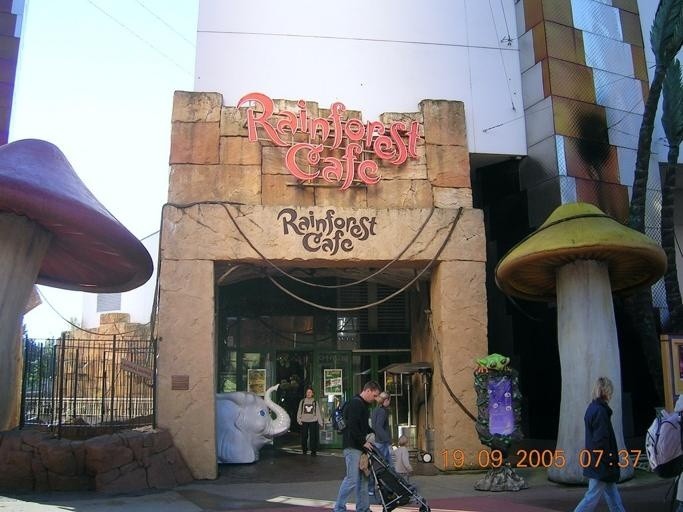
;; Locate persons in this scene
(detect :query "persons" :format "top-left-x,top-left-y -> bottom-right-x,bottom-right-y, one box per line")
572,375 -> 626,512
327,393 -> 339,421
392,434 -> 413,484
671,391 -> 683,511
296,387 -> 324,457
332,381 -> 382,512
368,392 -> 394,496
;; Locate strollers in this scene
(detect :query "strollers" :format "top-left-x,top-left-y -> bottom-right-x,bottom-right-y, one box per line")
363,444 -> 433,512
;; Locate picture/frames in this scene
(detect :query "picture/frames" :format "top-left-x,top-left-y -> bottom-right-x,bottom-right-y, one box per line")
323,368 -> 343,396
246,368 -> 267,397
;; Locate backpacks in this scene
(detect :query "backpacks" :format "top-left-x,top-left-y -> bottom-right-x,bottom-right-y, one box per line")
643,406 -> 682,504
328,396 -> 363,434
